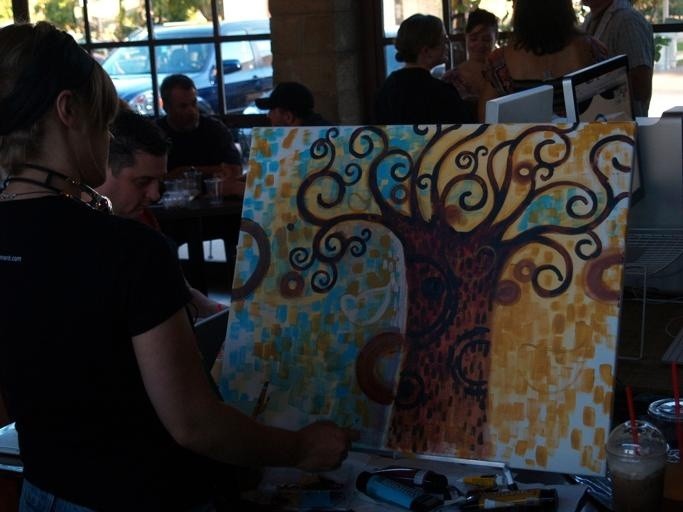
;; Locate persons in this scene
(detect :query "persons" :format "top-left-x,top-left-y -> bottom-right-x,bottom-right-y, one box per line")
442,9 -> 498,99
374,13 -> 478,124
255,81 -> 337,125
154,75 -> 241,165
482,0 -> 608,94
80,113 -> 230,319
578,0 -> 655,104
0,22 -> 362,512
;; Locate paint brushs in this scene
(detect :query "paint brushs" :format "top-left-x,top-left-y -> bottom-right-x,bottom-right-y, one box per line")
251,380 -> 270,420
342,419 -> 359,430
349,448 -> 401,459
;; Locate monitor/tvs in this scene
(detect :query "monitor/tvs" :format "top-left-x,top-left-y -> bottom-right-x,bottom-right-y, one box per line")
562,52 -> 646,211
483,83 -> 554,124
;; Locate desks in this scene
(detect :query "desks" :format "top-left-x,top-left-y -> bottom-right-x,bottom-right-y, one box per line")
149,165 -> 248,261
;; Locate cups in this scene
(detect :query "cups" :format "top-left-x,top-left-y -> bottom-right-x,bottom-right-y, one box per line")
662,450 -> 683,511
648,397 -> 683,449
604,419 -> 669,512
164,170 -> 225,207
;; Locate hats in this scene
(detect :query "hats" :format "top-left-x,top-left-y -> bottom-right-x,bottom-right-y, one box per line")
254,81 -> 314,110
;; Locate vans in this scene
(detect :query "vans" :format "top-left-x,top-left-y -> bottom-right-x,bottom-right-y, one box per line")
99,18 -> 275,117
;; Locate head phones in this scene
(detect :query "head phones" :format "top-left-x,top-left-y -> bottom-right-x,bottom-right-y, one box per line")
3,162 -> 114,215
